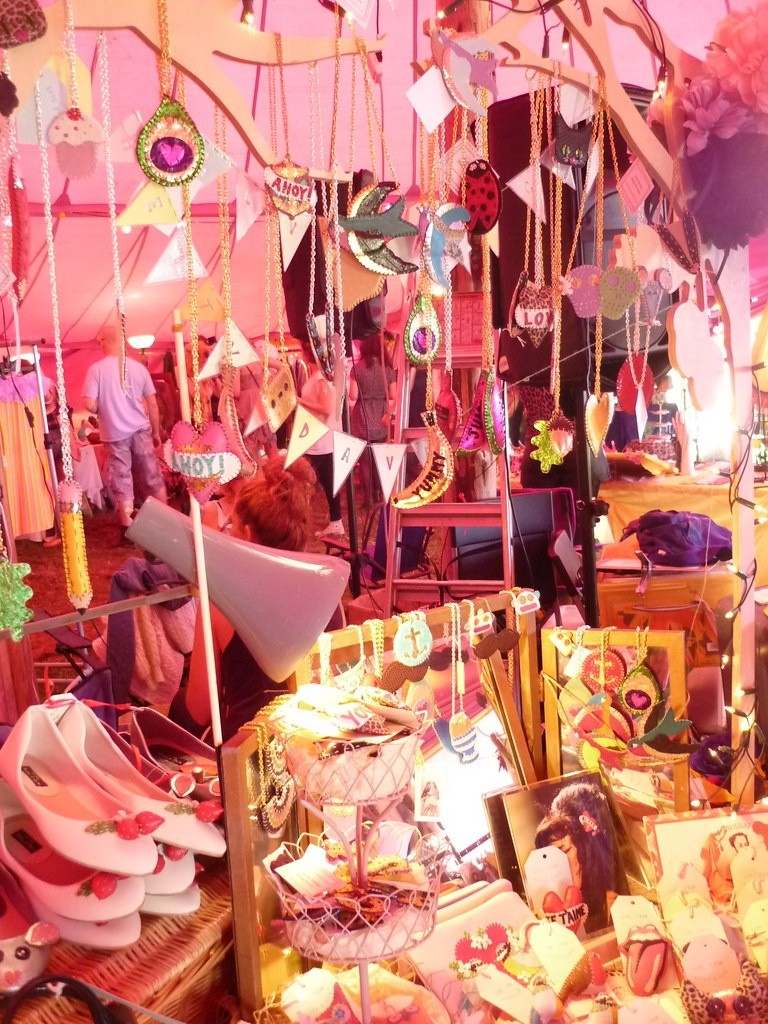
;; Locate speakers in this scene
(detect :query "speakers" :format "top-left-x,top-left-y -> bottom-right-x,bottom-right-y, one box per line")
487,76 -> 682,390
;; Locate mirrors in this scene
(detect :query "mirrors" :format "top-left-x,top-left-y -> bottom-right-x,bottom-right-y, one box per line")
292,608 -> 535,894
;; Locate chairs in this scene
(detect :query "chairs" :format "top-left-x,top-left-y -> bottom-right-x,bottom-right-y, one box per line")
320,487 -> 585,627
0,611 -> 116,751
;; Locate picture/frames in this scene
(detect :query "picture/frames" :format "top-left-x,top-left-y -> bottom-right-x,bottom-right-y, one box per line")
641,805 -> 768,999
223,694 -> 322,1024
484,770 -> 630,941
544,630 -> 690,821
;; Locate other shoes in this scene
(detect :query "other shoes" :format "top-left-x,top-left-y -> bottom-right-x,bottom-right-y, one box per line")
0,692 -> 225,999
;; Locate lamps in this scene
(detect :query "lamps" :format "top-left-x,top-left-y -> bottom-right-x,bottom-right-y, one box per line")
128,335 -> 155,352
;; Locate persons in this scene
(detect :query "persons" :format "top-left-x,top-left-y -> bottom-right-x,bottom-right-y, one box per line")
538,785 -> 621,934
0,334 -> 394,547
105,454 -> 330,748
604,375 -> 671,451
